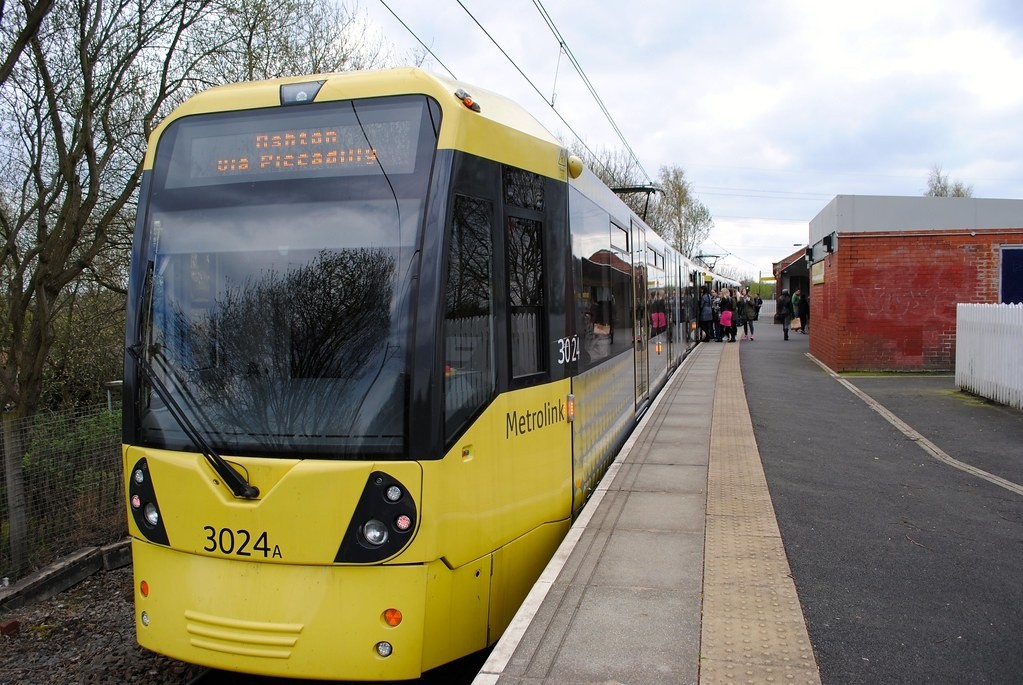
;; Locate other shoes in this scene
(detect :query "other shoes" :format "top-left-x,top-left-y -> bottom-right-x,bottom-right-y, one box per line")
702,340 -> 709,342
715,340 -> 722,342
729,340 -> 735,342
784,335 -> 788,340
750,335 -> 754,340
744,335 -> 747,340
800,330 -> 806,334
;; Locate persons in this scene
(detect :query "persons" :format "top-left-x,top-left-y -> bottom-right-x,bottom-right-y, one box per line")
700,286 -> 762,343
777,289 -> 795,341
607,294 -> 615,344
632,286 -> 695,351
583,312 -> 609,365
791,288 -> 810,335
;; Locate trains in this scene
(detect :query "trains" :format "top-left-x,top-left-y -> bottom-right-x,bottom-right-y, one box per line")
117,68 -> 745,685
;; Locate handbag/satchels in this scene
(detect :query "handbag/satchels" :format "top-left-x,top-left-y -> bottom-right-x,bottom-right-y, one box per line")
774,312 -> 782,320
791,318 -> 801,330
